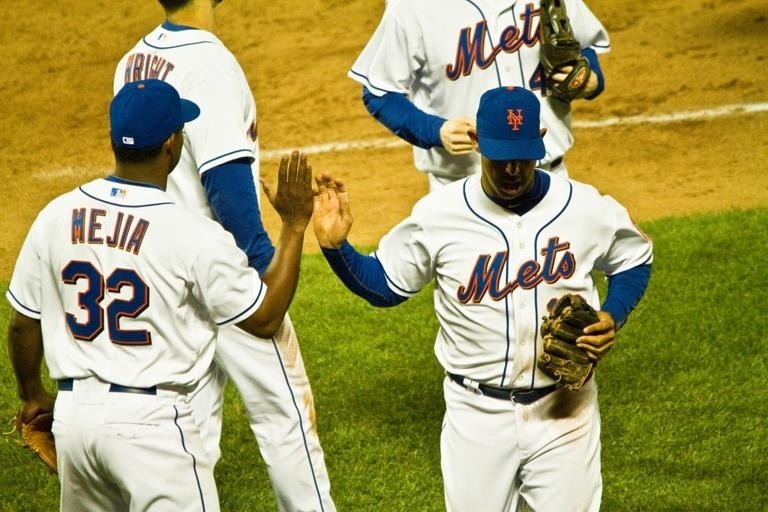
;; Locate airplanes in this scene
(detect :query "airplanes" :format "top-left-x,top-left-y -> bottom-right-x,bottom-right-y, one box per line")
2,409 -> 57,473
536,293 -> 600,391
539,0 -> 590,104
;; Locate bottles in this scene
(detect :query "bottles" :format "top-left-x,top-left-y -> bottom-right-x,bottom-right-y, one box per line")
109,79 -> 201,148
477,86 -> 546,162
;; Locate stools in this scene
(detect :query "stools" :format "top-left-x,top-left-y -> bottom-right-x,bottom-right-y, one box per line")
57,377 -> 158,395
447,371 -> 558,403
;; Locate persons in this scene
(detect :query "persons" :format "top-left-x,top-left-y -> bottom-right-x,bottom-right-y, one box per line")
110,0 -> 338,512
3,76 -> 314,512
343,0 -> 611,193
310,85 -> 654,512
